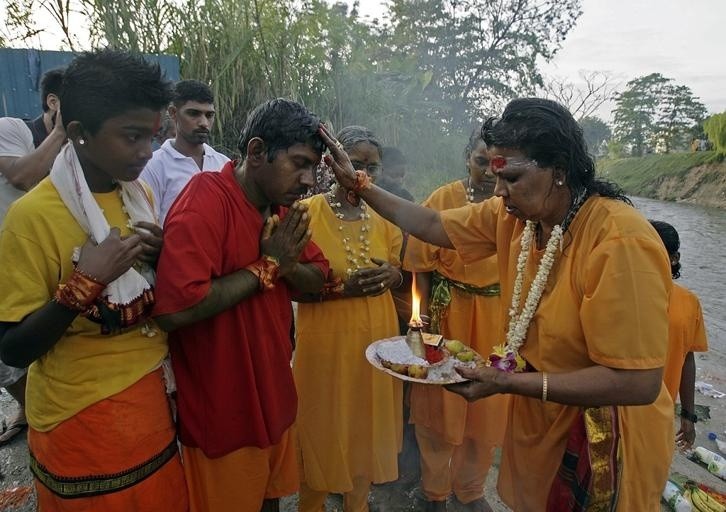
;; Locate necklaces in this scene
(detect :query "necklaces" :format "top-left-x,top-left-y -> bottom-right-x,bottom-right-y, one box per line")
465,176 -> 474,205
329,182 -> 370,281
485,187 -> 588,373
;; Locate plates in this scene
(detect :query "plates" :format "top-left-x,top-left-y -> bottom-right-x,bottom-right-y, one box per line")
362,332 -> 490,384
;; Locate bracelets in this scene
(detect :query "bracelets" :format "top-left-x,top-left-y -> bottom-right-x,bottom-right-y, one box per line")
345,170 -> 370,208
244,253 -> 280,292
320,267 -> 345,300
542,370 -> 549,404
391,271 -> 404,289
420,315 -> 431,325
680,408 -> 697,423
54,268 -> 106,312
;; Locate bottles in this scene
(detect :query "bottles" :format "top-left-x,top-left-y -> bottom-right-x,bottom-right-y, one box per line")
695,433 -> 726,479
404,321 -> 428,361
661,479 -> 690,508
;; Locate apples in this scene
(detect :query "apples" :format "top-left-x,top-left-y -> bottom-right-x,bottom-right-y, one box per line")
392,340 -> 475,379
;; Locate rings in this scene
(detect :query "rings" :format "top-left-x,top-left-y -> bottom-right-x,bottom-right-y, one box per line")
335,141 -> 344,152
380,283 -> 385,289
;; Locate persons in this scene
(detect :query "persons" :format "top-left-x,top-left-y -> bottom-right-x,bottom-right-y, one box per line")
150,98 -> 330,511
291,125 -> 404,512
373,146 -> 415,204
691,134 -> 707,152
0,47 -> 191,512
137,79 -> 232,231
402,129 -> 513,511
319,96 -> 676,511
647,218 -> 708,453
0,66 -> 70,444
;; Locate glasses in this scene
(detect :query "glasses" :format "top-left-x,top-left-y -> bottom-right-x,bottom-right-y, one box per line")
347,163 -> 383,178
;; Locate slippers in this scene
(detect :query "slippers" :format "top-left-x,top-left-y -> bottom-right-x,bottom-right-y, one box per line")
0,416 -> 30,446
